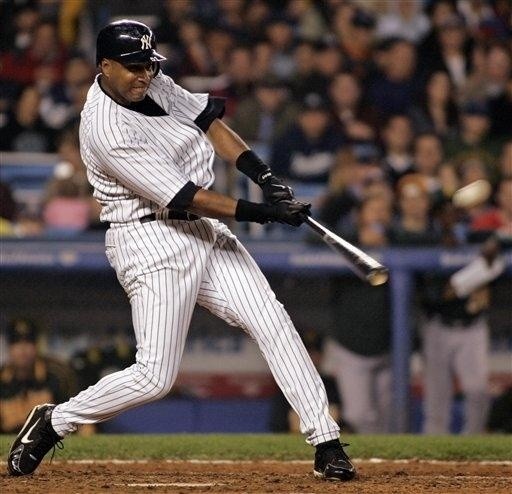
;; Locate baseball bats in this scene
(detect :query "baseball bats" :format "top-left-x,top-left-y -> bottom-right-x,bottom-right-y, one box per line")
303,213 -> 389,289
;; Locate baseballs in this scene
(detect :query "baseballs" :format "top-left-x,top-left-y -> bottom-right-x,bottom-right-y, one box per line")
453,179 -> 493,210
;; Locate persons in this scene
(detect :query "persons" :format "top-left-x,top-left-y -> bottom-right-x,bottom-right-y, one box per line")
7,20 -> 354,481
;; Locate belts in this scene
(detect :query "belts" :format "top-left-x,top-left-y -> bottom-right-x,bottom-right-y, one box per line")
139,211 -> 199,224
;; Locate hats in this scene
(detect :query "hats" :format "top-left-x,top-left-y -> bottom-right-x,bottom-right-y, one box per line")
96,20 -> 167,68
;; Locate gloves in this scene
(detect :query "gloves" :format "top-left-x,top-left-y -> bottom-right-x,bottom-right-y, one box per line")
236,150 -> 312,227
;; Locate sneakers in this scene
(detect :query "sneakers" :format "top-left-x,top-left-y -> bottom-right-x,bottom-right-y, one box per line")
313,438 -> 356,481
8,404 -> 63,476
